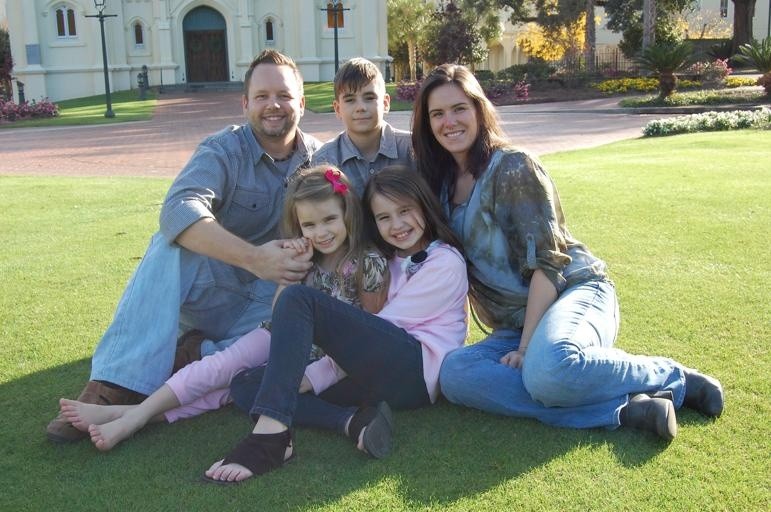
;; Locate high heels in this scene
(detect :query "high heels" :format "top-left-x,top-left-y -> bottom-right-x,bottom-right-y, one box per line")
627,391 -> 678,439
683,368 -> 723,417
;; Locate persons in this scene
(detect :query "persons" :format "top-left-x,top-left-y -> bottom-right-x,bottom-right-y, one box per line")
202,157 -> 472,486
308,55 -> 420,201
43,46 -> 326,446
409,58 -> 725,442
58,159 -> 393,456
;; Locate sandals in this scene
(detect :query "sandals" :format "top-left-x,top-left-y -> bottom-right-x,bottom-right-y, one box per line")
348,401 -> 393,459
203,430 -> 295,483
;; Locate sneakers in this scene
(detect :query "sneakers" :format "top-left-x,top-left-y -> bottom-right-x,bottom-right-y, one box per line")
46,381 -> 137,443
172,329 -> 208,375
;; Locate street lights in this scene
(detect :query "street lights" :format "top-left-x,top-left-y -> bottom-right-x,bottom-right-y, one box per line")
330,0 -> 341,76
92,0 -> 116,118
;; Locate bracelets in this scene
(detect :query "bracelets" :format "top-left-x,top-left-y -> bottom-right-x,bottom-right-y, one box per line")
517,346 -> 527,352
